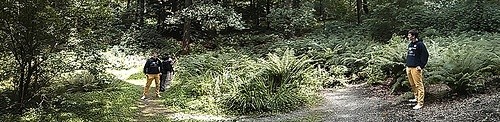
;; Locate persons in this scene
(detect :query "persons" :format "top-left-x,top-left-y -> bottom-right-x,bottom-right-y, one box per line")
143,51 -> 163,100
405,29 -> 428,110
157,53 -> 176,92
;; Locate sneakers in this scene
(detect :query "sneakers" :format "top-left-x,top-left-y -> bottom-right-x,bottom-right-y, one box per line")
413,103 -> 424,110
409,98 -> 418,102
156,94 -> 162,98
141,95 -> 146,100
